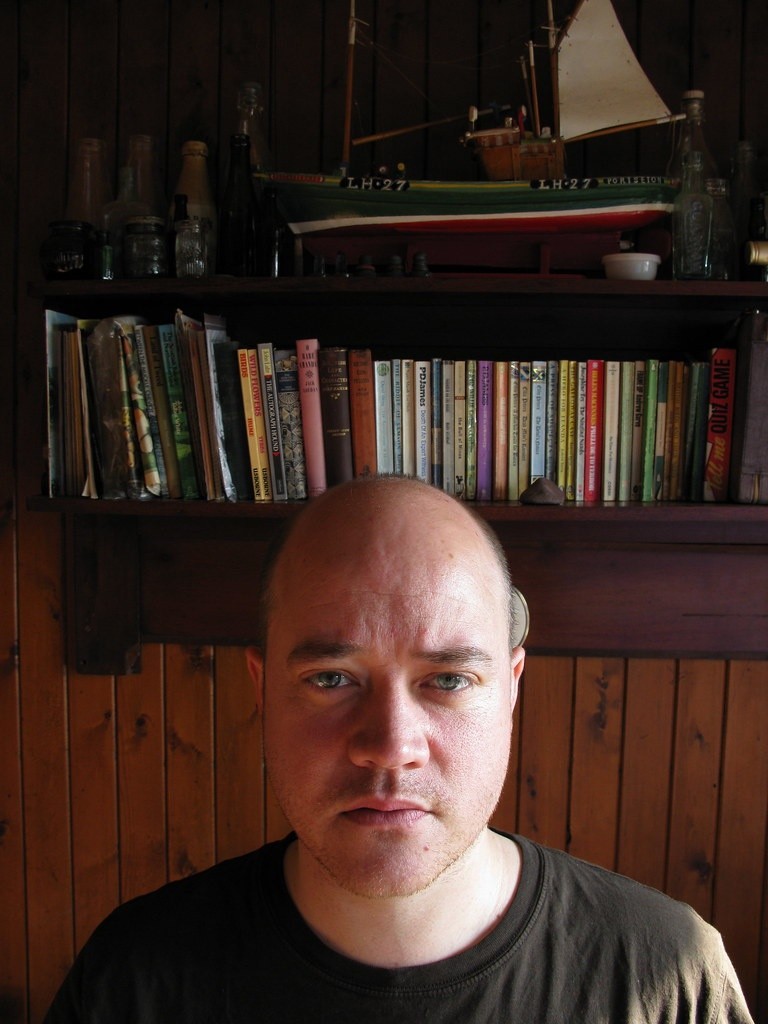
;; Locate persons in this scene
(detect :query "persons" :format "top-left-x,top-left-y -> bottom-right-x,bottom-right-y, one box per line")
40,473 -> 754,1024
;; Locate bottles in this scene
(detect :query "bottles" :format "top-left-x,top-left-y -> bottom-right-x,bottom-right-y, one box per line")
111,133 -> 167,276
55,136 -> 120,282
672,88 -> 721,279
169,141 -> 221,278
215,83 -> 275,276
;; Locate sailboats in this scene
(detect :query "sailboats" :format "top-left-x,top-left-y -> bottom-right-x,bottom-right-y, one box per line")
259,1 -> 688,273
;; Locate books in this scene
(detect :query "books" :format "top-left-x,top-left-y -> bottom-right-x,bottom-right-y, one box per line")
46,309 -> 735,502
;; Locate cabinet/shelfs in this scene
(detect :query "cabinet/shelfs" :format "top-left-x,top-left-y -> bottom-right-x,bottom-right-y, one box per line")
22,278 -> 768,675
